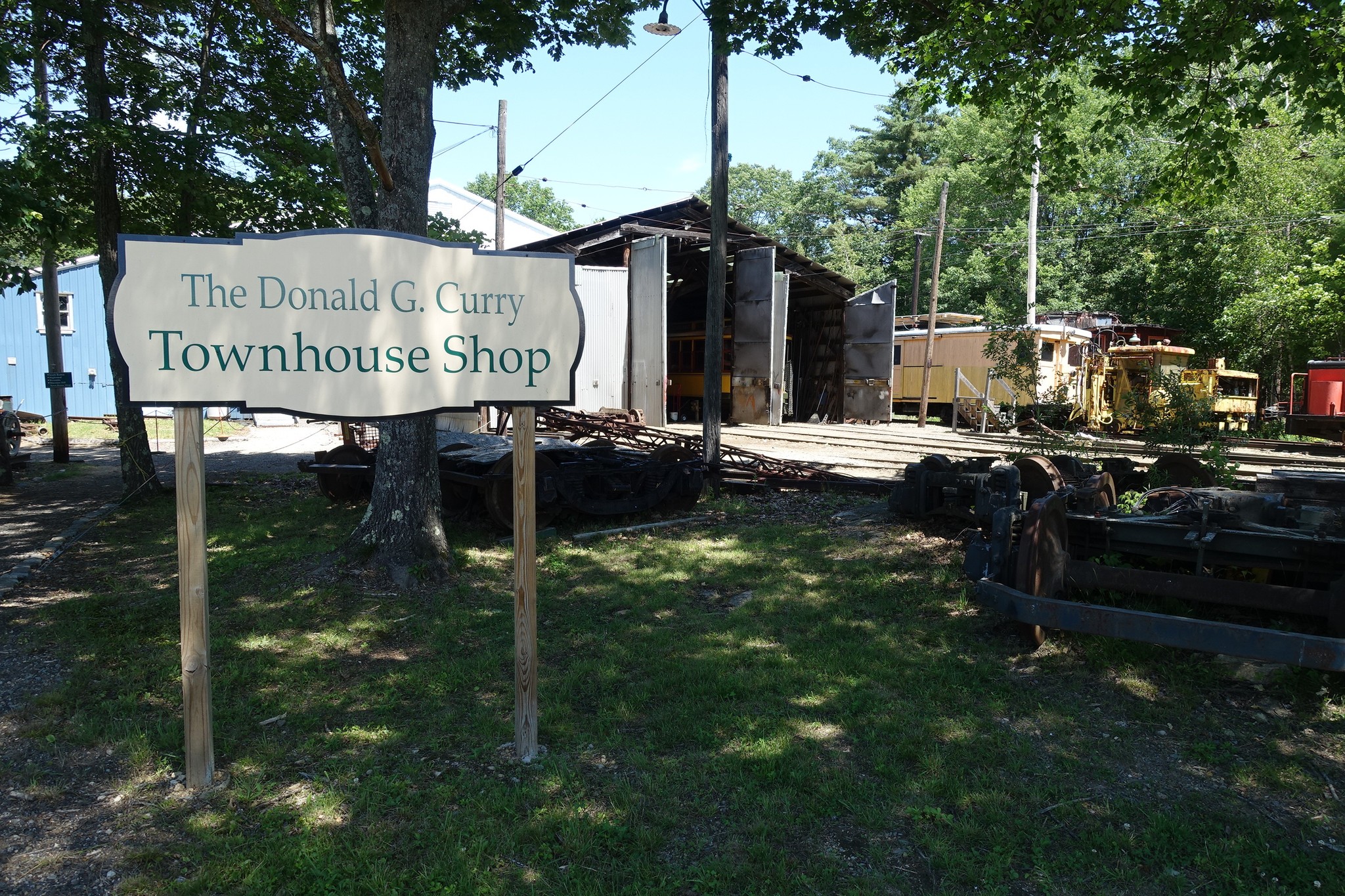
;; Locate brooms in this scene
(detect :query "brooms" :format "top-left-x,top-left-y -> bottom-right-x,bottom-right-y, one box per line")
807,383 -> 827,423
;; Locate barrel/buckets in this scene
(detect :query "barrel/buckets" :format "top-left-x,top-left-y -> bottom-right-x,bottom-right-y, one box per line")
670,412 -> 678,422
